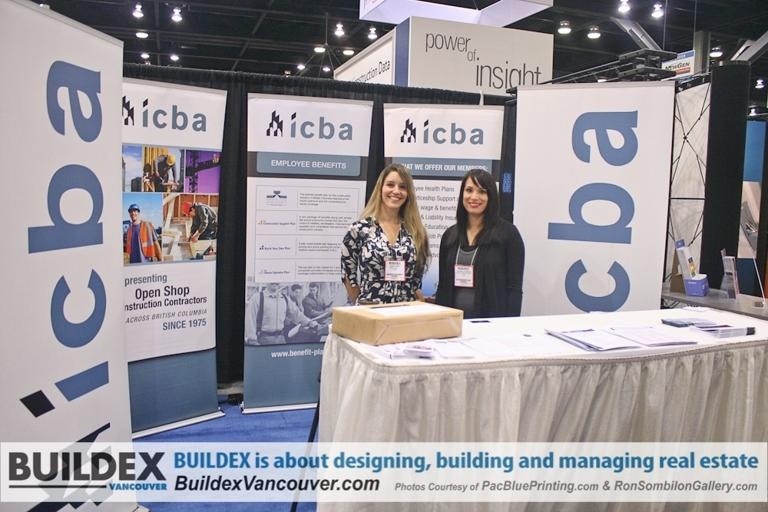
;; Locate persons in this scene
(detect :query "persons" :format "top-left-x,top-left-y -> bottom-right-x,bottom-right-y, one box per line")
341,165 -> 432,305
140,154 -> 179,193
179,200 -> 218,258
125,203 -> 162,263
245,282 -> 330,346
434,169 -> 525,318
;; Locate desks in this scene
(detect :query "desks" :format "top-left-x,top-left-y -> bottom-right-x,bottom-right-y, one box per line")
288,282 -> 768,511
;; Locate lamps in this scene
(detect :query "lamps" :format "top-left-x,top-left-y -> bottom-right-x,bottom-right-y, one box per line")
556,20 -> 603,40
131,2 -> 183,23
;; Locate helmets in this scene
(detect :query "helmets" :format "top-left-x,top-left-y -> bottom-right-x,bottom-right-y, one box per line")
128,204 -> 141,212
165,155 -> 176,166
182,202 -> 193,215
